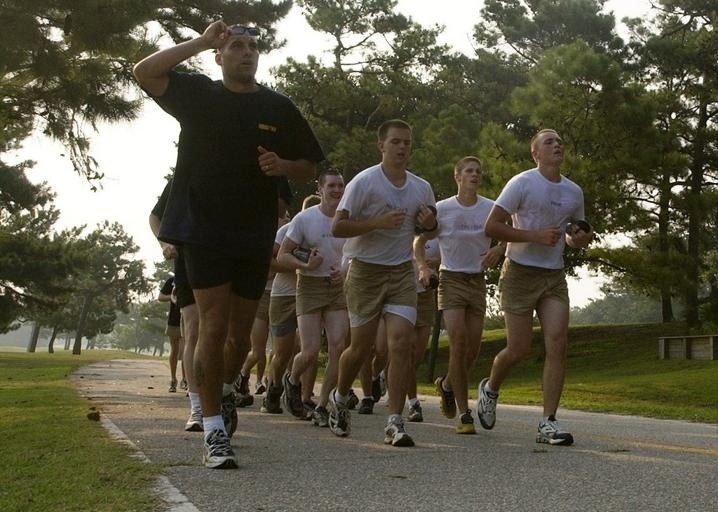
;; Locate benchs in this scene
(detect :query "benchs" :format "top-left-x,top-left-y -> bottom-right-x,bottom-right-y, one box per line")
658,334 -> 718,360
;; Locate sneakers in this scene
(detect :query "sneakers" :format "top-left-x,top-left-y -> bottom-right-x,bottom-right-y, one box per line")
435,377 -> 499,433
169,369 -> 386,436
536,415 -> 573,445
408,401 -> 422,421
202,429 -> 238,468
385,422 -> 414,446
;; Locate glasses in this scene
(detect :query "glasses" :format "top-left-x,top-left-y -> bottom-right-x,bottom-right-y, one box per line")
230,26 -> 260,35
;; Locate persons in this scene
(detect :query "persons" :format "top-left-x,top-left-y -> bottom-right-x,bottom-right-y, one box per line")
148,167 -> 210,435
477,127 -> 593,447
233,119 -> 511,446
131,18 -> 326,469
